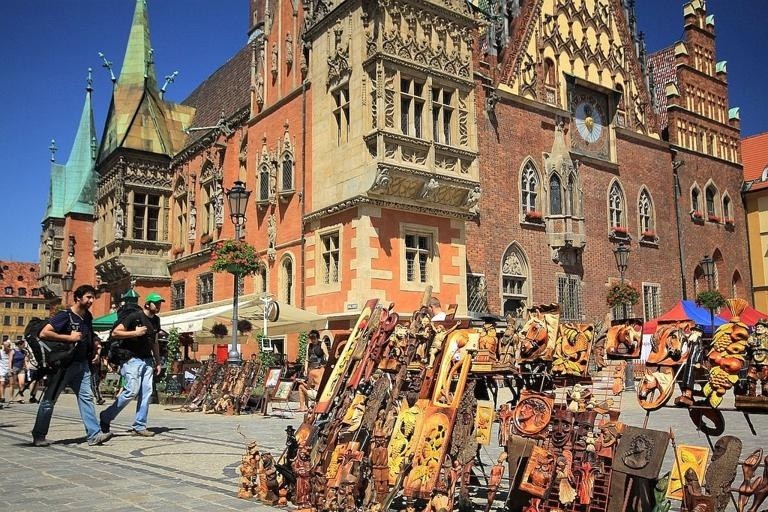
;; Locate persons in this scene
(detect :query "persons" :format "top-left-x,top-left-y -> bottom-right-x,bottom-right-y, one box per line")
239,422 -> 509,507
0,334 -> 38,408
414,319 -> 463,369
429,297 -> 447,321
294,330 -> 329,412
250,354 -> 258,360
747,320 -> 768,396
31,285 -> 166,447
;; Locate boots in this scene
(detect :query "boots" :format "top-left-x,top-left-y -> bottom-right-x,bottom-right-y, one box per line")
29,394 -> 37,402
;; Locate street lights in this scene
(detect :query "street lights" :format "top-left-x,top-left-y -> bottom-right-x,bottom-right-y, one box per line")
224,180 -> 253,364
611,239 -> 634,391
699,254 -> 716,338
60,271 -> 77,310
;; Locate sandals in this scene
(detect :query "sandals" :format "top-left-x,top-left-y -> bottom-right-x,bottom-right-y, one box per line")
9,400 -> 25,404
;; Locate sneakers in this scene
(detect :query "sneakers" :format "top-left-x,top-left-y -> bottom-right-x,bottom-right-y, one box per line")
98,399 -> 105,404
0,398 -> 5,402
132,429 -> 154,436
34,436 -> 48,446
88,411 -> 113,446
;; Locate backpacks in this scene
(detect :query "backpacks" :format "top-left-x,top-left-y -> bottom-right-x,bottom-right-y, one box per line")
22,317 -> 73,383
107,304 -> 143,367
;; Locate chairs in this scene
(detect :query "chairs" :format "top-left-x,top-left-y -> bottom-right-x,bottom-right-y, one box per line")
267,379 -> 297,420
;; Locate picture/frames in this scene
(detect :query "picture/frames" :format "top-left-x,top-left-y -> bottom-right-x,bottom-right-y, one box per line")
261,365 -> 292,402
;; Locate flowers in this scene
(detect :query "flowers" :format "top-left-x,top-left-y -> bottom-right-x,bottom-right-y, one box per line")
606,280 -> 640,310
207,241 -> 267,278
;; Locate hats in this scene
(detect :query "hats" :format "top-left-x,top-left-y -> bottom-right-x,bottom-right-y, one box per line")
308,330 -> 320,339
146,293 -> 165,304
306,355 -> 321,363
